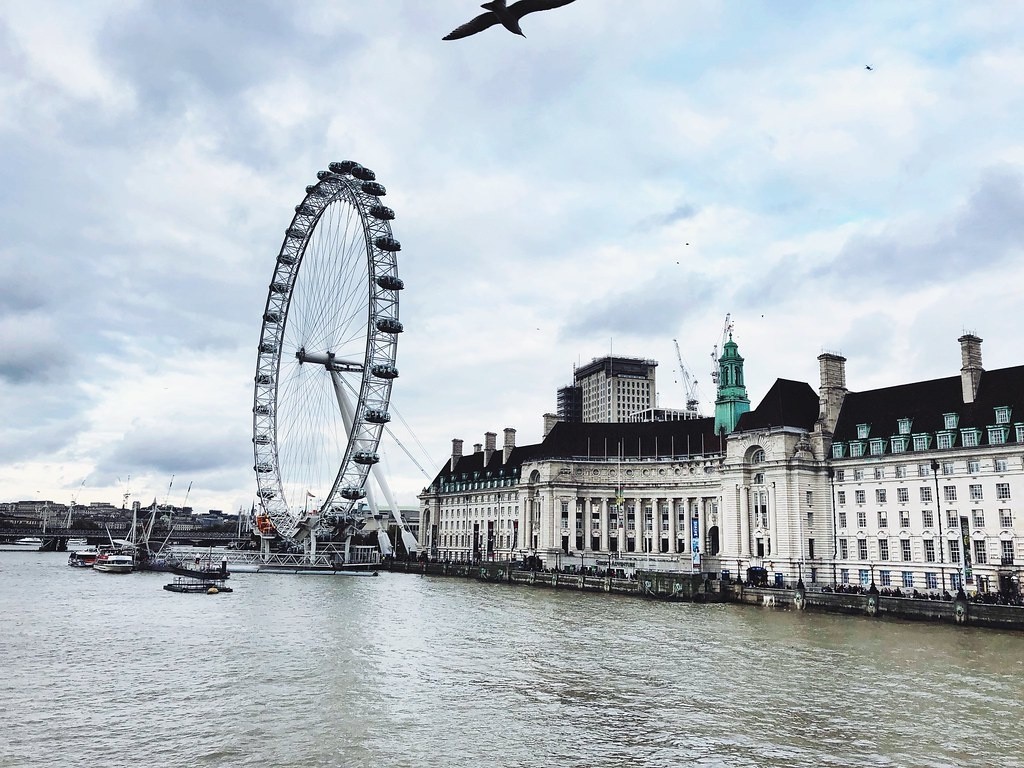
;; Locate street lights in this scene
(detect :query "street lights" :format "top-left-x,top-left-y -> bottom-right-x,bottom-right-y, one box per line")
608,554 -> 611,569
955,564 -> 966,600
555,552 -> 559,570
736,558 -> 743,582
868,561 -> 879,594
408,544 -> 411,565
796,559 -> 805,589
581,553 -> 584,567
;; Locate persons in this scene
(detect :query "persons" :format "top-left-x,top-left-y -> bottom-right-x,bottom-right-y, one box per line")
880,586 -> 952,601
820,583 -> 867,595
530,564 -> 637,580
967,591 -> 1024,606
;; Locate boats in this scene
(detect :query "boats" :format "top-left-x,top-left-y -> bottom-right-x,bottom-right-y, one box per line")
68,551 -> 97,567
0,501 -> 97,551
93,544 -> 135,573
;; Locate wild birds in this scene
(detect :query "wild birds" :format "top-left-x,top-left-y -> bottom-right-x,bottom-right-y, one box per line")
866,65 -> 873,71
442,0 -> 572,42
